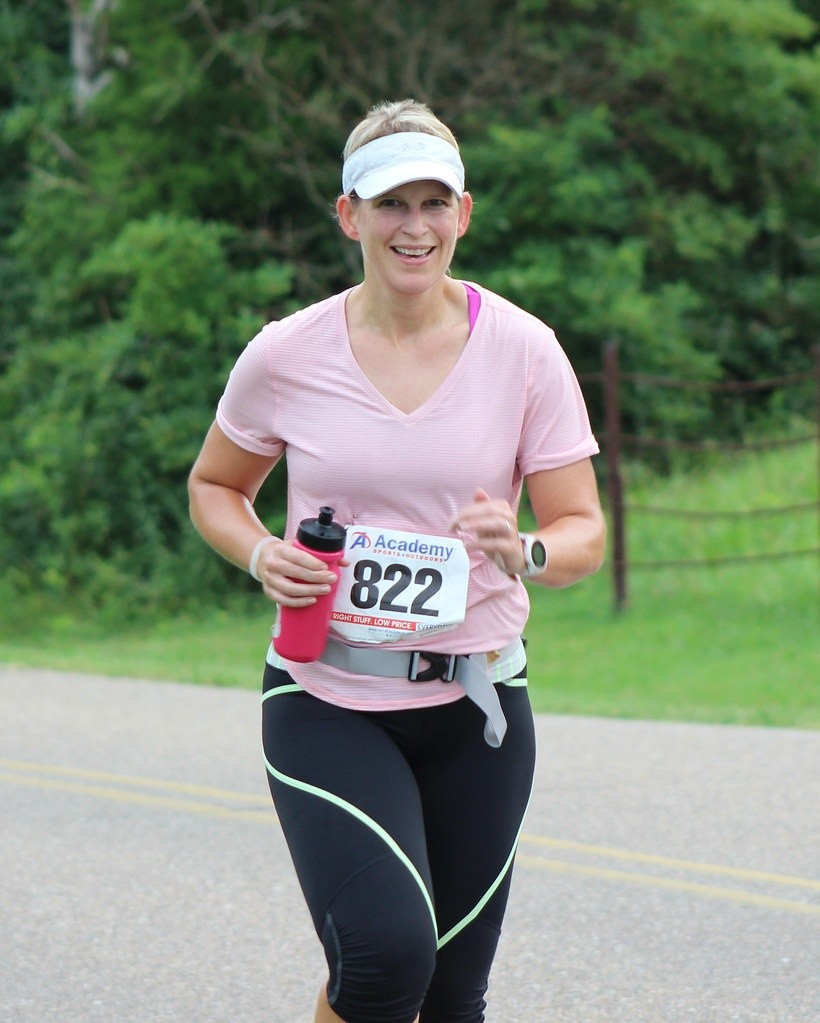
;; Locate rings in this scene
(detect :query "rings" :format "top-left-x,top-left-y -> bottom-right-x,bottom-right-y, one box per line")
505,520 -> 510,531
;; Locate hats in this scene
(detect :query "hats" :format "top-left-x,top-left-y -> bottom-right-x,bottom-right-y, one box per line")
341,131 -> 466,200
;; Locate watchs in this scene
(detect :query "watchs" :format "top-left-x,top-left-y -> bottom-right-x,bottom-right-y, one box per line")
518,532 -> 548,577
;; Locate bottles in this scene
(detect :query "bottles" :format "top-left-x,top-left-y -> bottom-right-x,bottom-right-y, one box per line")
272,506 -> 347,664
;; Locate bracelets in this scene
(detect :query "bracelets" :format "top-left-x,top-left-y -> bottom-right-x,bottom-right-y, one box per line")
250,536 -> 279,582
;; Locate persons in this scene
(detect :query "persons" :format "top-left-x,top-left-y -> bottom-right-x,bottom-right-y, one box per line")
186,100 -> 609,1023
